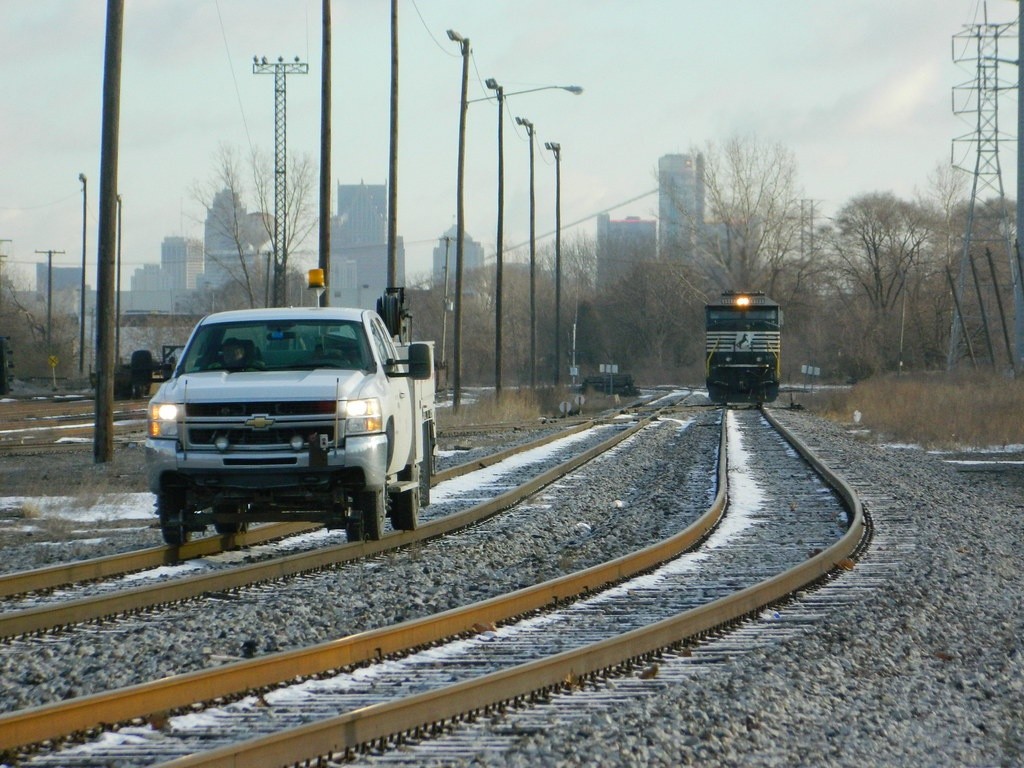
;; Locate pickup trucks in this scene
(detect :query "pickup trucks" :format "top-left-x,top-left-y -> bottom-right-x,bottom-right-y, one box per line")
138,268 -> 438,547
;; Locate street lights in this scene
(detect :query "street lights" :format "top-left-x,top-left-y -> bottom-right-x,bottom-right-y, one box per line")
76,172 -> 88,376
544,139 -> 563,388
453,83 -> 583,415
484,78 -> 507,412
515,115 -> 537,395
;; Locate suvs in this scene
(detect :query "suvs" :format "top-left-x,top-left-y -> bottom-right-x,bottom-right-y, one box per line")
115,192 -> 123,375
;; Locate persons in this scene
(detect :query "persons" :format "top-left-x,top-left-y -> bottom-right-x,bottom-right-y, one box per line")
207,338 -> 265,370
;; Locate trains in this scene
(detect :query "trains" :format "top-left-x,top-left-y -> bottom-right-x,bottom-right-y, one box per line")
705,288 -> 783,409
